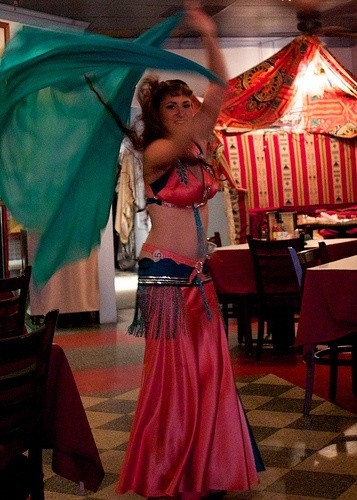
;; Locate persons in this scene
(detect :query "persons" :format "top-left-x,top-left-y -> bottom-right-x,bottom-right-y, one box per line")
82,1 -> 266,500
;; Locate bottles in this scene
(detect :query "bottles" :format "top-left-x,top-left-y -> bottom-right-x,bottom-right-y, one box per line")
272,220 -> 278,241
261,221 -> 268,241
280,221 -> 286,240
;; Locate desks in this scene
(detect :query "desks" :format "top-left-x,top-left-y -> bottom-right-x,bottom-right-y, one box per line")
209,236 -> 357,417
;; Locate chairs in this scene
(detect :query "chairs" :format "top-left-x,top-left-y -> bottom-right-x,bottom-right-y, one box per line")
207,232 -> 244,345
0,261 -> 59,500
246,234 -> 334,359
